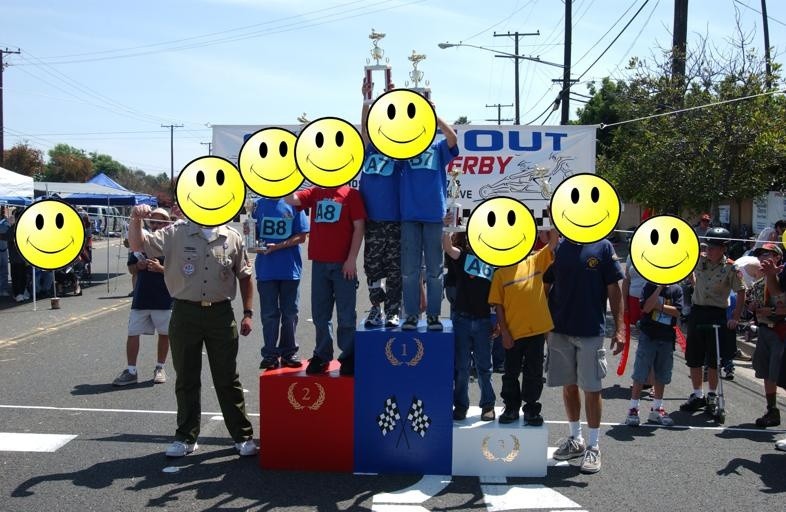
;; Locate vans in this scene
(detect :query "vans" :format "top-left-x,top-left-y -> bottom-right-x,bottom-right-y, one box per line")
82,205 -> 123,235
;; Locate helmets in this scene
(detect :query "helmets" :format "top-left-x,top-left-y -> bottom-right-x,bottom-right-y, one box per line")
700,227 -> 730,247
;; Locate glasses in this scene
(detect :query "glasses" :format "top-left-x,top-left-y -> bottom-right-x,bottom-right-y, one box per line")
150,221 -> 162,224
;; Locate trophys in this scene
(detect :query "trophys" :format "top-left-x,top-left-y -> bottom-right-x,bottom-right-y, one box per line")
406,49 -> 430,93
532,162 -> 555,230
361,25 -> 398,107
442,164 -> 467,233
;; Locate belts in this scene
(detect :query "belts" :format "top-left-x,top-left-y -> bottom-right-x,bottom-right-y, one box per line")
185,300 -> 226,307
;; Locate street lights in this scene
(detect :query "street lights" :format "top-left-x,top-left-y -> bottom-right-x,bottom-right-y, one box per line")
439,39 -> 566,126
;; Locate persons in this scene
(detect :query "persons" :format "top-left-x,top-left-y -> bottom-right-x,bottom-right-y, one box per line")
127,201 -> 260,461
621,212 -> 786,379
441,210 -> 498,422
487,204 -> 562,427
0,203 -> 95,305
541,234 -> 630,475
242,196 -> 311,370
399,99 -> 460,333
758,256 -> 786,451
285,185 -> 368,376
744,242 -> 786,429
110,207 -> 174,387
678,225 -> 749,420
359,78 -> 403,329
623,281 -> 686,428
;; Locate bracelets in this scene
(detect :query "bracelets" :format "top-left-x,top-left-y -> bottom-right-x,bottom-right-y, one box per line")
771,306 -> 777,317
244,308 -> 254,317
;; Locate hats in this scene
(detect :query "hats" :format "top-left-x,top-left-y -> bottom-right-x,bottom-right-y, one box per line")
151,208 -> 170,220
753,243 -> 783,257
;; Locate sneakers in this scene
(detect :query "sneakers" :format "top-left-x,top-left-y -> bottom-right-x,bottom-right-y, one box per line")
453,405 -> 543,426
234,441 -> 257,456
756,407 -> 786,450
725,347 -> 751,377
625,407 -> 673,426
553,436 -> 602,473
259,353 -> 354,375
113,369 -> 137,385
364,306 -> 443,330
153,369 -> 166,383
166,440 -> 198,457
680,392 -> 719,411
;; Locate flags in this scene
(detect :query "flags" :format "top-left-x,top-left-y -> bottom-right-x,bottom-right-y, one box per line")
373,395 -> 400,437
407,397 -> 433,439
